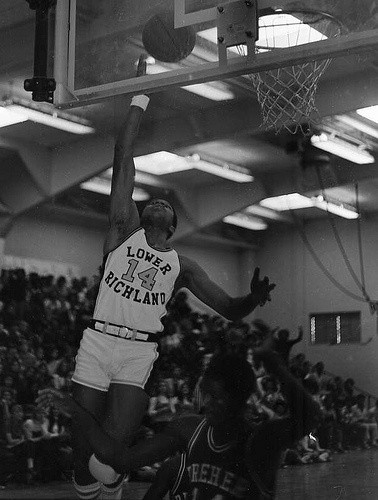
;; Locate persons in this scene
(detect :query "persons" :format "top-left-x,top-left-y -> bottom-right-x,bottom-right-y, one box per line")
69,55 -> 277,500
35,346 -> 322,500
0,267 -> 378,490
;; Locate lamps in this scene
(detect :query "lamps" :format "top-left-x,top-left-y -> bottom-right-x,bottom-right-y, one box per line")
312,195 -> 359,219
312,132 -> 376,165
223,212 -> 268,231
186,153 -> 254,184
136,57 -> 235,102
0,96 -> 96,135
81,176 -> 150,201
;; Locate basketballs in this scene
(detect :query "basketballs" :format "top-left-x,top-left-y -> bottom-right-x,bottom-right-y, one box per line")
142,11 -> 196,63
